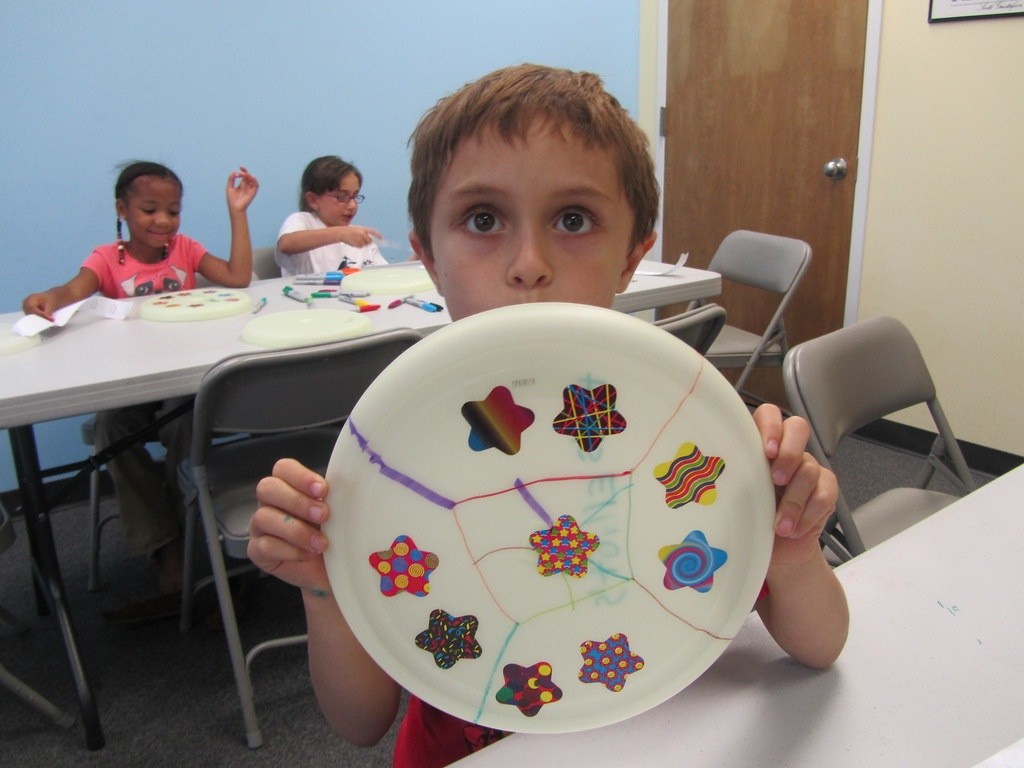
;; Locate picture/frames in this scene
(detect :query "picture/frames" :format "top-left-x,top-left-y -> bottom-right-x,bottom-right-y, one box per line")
928,0 -> 1024,23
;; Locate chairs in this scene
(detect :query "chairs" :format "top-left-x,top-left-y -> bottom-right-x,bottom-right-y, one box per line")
782,314 -> 975,568
175,228 -> 813,753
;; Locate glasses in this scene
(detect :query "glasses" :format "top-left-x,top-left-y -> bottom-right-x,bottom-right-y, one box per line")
321,189 -> 365,207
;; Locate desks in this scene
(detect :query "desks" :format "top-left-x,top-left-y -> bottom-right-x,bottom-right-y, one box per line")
0,259 -> 722,749
434,458 -> 1024,768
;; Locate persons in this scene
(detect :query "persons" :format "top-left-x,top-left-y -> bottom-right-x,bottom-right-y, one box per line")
274,155 -> 421,279
20,161 -> 260,600
247,62 -> 850,768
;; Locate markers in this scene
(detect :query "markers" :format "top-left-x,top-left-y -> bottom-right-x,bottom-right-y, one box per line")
252,297 -> 266,314
339,289 -> 380,312
282,286 -> 338,305
406,298 -> 443,313
293,272 -> 343,285
388,295 -> 415,309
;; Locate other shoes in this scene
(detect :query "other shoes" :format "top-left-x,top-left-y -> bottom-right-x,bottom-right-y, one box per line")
161,586 -> 247,632
